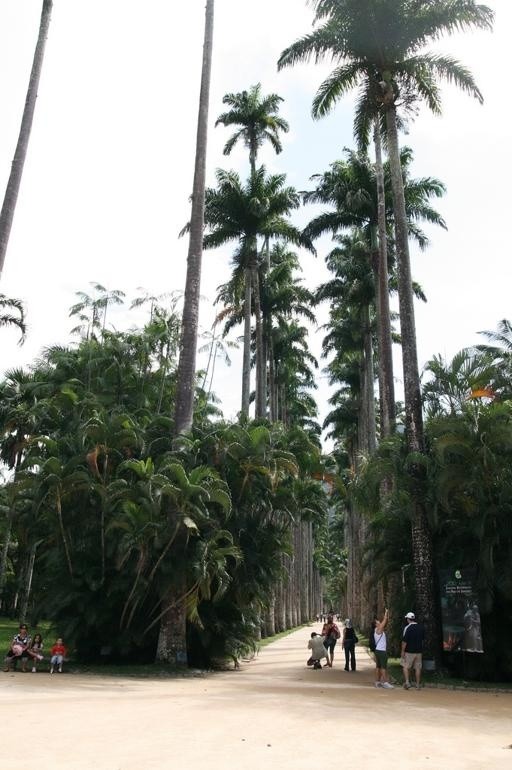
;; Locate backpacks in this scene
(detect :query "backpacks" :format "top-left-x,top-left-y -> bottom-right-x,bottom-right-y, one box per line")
327,624 -> 337,639
368,627 -> 383,651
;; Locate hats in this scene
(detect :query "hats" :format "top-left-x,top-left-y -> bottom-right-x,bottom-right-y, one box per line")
404,611 -> 415,619
343,619 -> 352,625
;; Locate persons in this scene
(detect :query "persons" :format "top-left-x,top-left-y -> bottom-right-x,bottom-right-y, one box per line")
398,612 -> 423,690
2,623 -> 32,673
316,609 -> 341,622
371,607 -> 394,689
50,637 -> 65,674
321,616 -> 338,668
464,608 -> 473,632
26,633 -> 43,672
306,631 -> 327,669
467,604 -> 483,653
341,618 -> 357,672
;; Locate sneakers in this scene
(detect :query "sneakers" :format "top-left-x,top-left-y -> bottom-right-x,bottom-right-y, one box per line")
314,662 -> 355,672
36,655 -> 43,660
374,680 -> 421,691
4,668 -> 63,674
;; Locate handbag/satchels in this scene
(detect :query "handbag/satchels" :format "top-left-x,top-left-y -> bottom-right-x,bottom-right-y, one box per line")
353,635 -> 358,643
